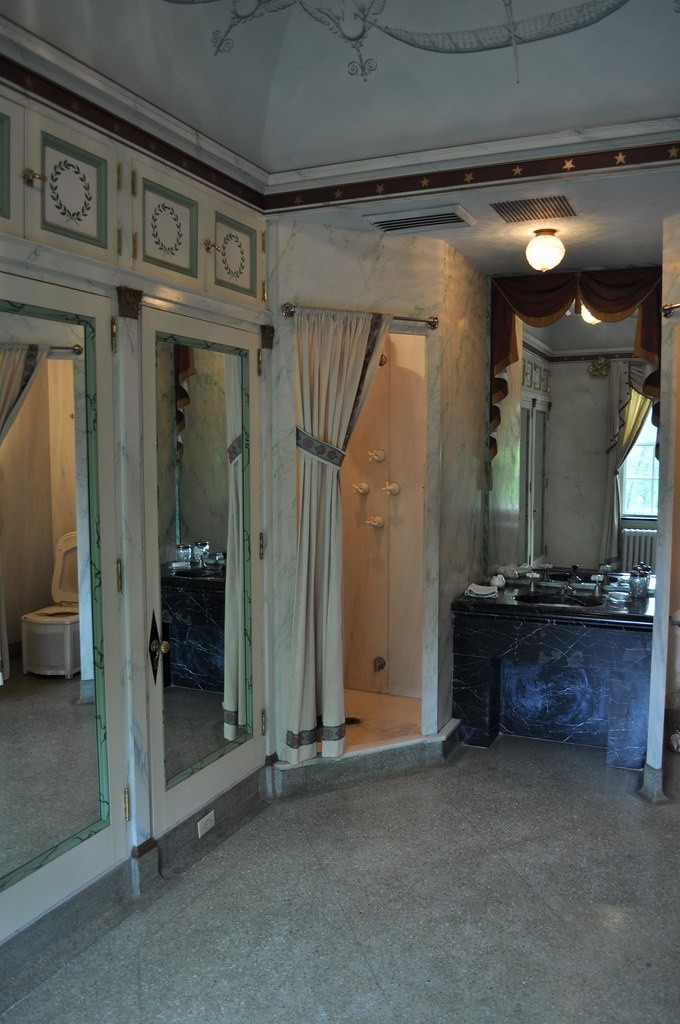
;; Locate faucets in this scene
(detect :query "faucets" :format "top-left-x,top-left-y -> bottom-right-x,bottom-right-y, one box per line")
195,551 -> 209,568
554,582 -> 579,598
567,564 -> 582,585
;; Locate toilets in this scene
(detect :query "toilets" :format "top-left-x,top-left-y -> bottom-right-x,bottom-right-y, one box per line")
19,529 -> 81,681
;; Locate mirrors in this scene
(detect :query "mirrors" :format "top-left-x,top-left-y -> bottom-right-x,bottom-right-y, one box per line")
521,404 -> 528,568
2,299 -> 114,888
535,408 -> 545,561
520,311 -> 662,573
152,329 -> 252,794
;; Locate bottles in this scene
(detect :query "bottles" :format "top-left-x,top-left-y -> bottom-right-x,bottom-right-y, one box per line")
174,545 -> 192,561
193,542 -> 210,560
628,566 -> 648,598
632,561 -> 652,587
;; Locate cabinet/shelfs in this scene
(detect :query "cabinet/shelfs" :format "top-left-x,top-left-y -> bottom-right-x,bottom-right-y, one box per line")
0,271 -> 132,947
518,351 -> 552,572
141,302 -> 271,840
1,94 -> 122,268
131,156 -> 269,310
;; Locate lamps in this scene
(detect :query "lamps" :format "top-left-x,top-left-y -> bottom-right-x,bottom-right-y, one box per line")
525,228 -> 566,273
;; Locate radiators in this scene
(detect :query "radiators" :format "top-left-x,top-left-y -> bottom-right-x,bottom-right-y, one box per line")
621,528 -> 658,574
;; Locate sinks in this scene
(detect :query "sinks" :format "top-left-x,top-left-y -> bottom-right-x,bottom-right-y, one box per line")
169,565 -> 230,580
549,569 -> 619,586
511,590 -> 608,610
